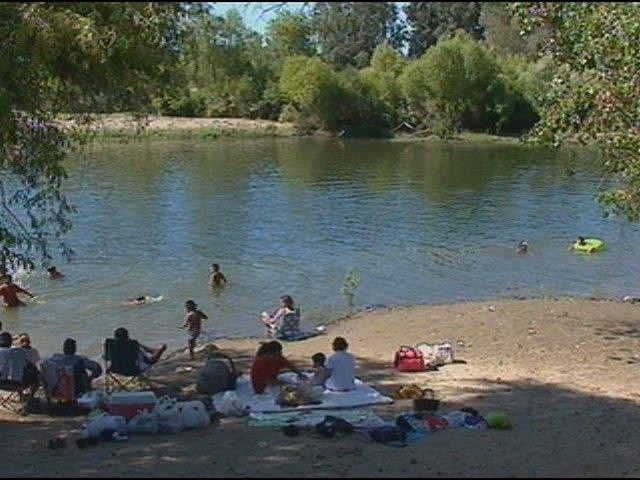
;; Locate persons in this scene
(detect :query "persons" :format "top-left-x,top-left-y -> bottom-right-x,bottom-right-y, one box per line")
321,335 -> 357,393
35,336 -> 103,401
257,294 -> 296,330
207,263 -> 227,285
513,238 -> 529,257
46,266 -> 65,281
117,293 -> 164,307
302,351 -> 332,387
101,326 -> 167,374
11,333 -> 40,370
0,331 -> 40,400
568,235 -> 596,254
248,339 -> 327,398
176,299 -> 209,360
0,281 -> 35,309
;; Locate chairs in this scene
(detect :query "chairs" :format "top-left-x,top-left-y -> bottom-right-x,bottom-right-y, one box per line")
0,340 -> 152,416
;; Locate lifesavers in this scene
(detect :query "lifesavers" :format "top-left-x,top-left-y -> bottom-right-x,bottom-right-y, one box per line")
574,238 -> 607,253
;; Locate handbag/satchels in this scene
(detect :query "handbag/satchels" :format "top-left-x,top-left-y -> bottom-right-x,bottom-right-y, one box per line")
414,389 -> 439,411
395,346 -> 424,371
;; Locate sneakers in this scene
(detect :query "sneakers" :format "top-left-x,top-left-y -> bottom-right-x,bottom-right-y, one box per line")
76,429 -> 128,447
